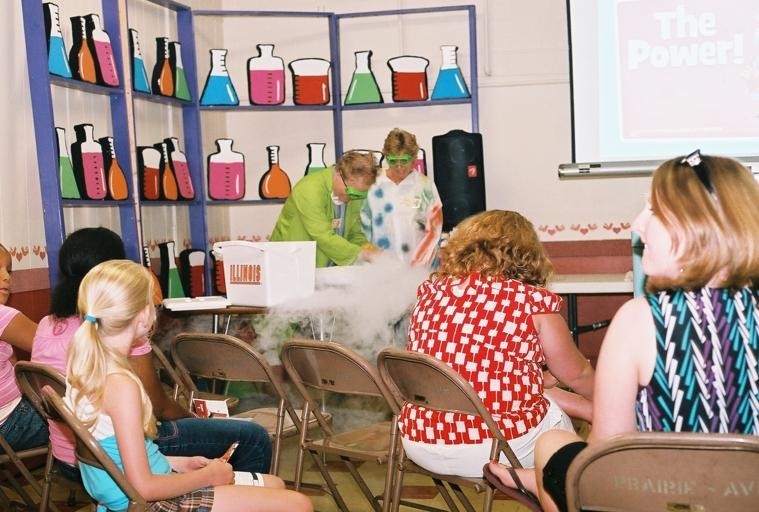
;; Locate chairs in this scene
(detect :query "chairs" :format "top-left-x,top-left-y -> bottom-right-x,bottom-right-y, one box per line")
565,433 -> 757,512
170,333 -> 351,512
280,339 -> 458,512
43,385 -> 156,512
15,361 -> 80,508
1,437 -> 58,508
149,342 -> 238,420
376,348 -> 526,511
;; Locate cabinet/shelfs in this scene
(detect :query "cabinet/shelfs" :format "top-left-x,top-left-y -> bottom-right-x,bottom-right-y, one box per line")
544,296 -> 634,361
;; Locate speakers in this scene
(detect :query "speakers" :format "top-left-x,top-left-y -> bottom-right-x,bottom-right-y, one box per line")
431,130 -> 486,233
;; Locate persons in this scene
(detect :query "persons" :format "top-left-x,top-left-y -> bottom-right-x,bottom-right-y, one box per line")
269,130 -> 444,273
489,150 -> 759,512
398,210 -> 595,477
0,243 -> 50,455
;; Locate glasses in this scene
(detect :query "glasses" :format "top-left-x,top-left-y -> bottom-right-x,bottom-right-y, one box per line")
678,148 -> 717,208
386,153 -> 414,167
340,174 -> 369,200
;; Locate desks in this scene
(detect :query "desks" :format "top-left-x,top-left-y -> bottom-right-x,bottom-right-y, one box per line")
165,304 -> 274,334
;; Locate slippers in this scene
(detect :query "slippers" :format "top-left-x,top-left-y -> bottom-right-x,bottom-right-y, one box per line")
483,462 -> 543,512
